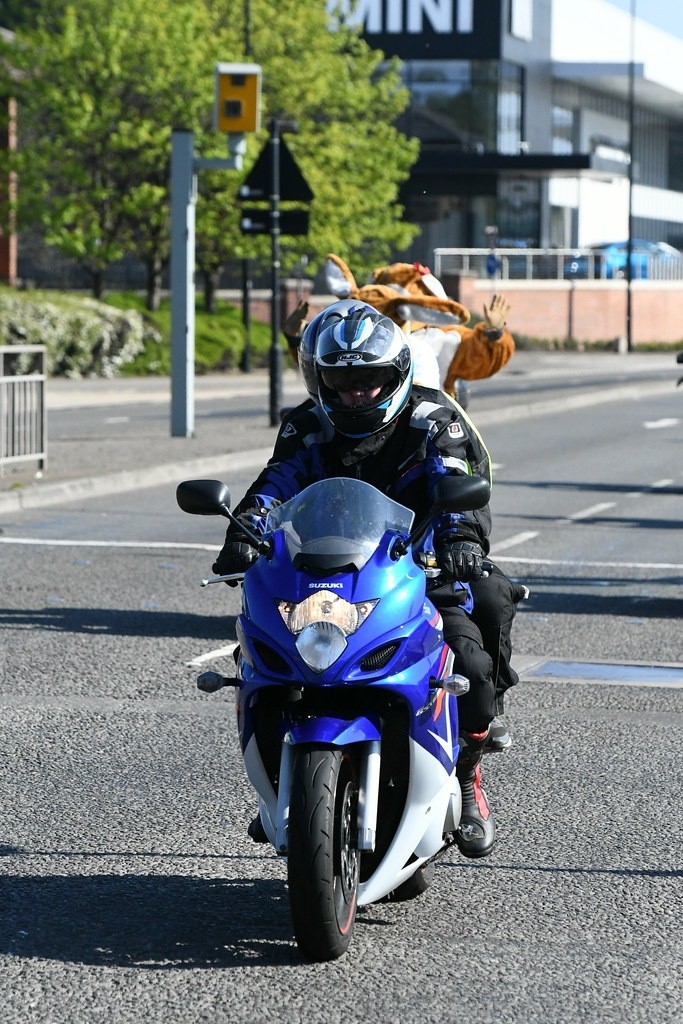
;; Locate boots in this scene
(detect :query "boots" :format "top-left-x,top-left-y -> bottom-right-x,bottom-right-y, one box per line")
452,725 -> 496,860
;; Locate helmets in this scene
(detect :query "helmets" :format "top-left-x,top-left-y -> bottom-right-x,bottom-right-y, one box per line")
316,312 -> 414,437
298,299 -> 383,407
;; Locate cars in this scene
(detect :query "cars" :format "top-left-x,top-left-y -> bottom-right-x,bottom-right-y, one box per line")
563,238 -> 679,280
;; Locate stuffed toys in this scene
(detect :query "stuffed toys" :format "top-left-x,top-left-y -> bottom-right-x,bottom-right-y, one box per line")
321,256 -> 516,401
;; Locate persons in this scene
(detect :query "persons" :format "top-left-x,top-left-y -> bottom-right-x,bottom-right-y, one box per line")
270,298 -> 529,751
213,318 -> 503,857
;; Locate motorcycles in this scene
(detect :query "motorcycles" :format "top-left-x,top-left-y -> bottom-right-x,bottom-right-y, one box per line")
176,472 -> 504,963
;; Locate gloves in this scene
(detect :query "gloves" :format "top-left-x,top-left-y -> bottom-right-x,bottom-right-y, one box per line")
435,530 -> 483,581
217,529 -> 261,587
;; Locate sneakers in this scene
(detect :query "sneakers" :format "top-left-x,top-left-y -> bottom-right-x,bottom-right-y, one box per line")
490,718 -> 512,750
